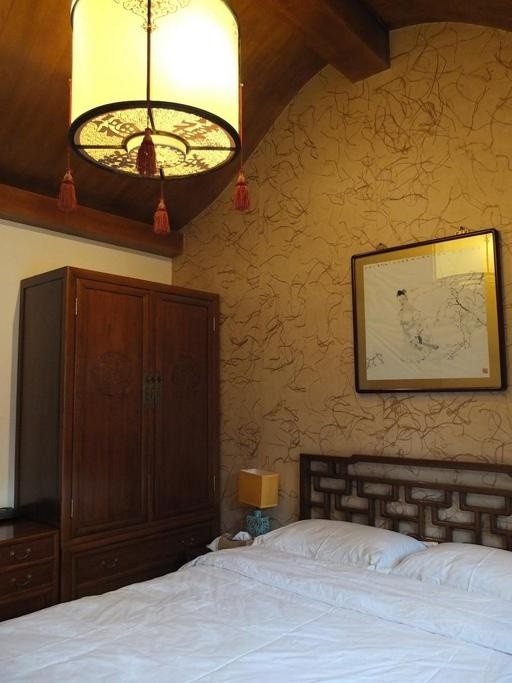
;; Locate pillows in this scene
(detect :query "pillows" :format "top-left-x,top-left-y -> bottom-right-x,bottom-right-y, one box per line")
390,542 -> 512,601
252,519 -> 438,575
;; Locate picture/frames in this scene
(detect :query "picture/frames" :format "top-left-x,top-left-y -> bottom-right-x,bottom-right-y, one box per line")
351,226 -> 507,393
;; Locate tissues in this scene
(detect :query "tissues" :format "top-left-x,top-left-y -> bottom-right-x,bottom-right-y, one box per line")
218,531 -> 255,551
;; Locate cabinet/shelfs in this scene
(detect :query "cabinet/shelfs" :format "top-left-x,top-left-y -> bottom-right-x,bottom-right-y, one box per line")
0,520 -> 59,623
14,266 -> 220,602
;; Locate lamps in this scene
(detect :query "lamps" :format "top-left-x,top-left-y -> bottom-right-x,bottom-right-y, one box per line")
238,468 -> 279,537
58,0 -> 250,238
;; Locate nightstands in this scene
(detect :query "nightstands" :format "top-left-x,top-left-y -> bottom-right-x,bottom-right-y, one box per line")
178,546 -> 212,569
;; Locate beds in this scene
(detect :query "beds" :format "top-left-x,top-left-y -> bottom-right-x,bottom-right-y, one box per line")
0,454 -> 512,682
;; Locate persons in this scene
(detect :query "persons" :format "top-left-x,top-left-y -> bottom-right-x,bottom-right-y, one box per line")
396,288 -> 439,356
